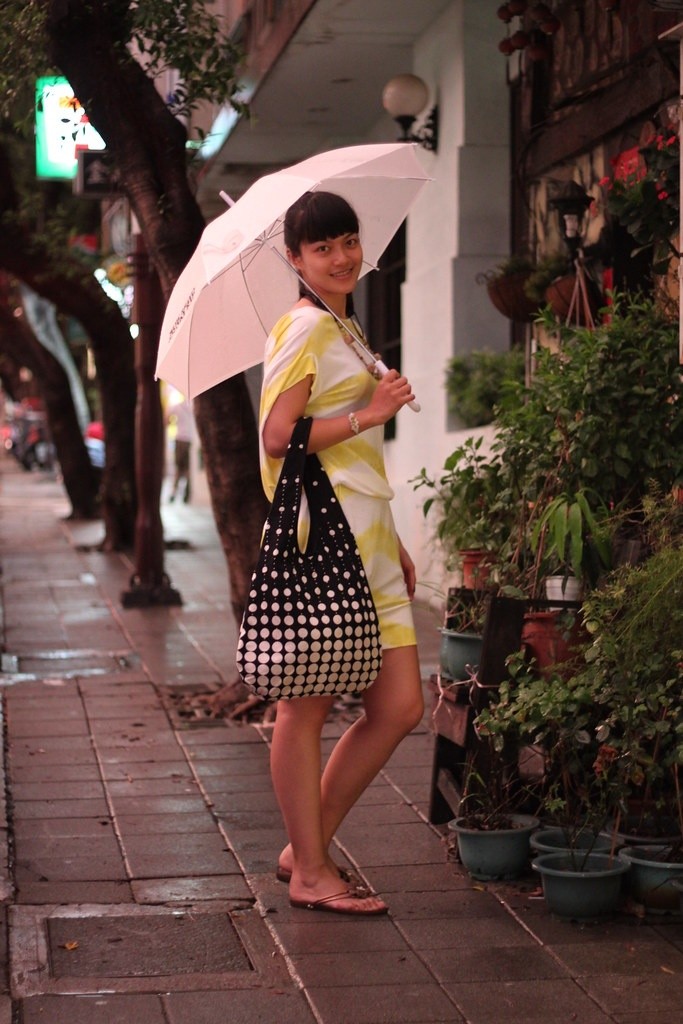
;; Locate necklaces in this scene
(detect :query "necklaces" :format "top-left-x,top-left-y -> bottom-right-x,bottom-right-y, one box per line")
333,317 -> 383,382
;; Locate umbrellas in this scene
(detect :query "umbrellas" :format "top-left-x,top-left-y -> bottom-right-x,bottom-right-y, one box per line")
153,145 -> 436,413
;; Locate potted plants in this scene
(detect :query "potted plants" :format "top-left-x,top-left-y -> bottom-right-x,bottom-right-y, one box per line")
413,123 -> 682,924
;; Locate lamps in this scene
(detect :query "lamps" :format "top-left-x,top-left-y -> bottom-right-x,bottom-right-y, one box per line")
382,73 -> 439,154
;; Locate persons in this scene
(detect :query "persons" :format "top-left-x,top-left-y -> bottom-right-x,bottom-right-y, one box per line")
164,401 -> 195,503
257,192 -> 428,915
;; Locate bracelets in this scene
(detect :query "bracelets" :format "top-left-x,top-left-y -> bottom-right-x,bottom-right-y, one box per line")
348,413 -> 360,435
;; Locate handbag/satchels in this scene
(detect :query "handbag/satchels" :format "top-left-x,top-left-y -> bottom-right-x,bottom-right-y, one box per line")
234,416 -> 382,699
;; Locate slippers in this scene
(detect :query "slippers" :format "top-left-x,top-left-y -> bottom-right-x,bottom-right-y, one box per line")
289,883 -> 388,916
276,865 -> 359,883
